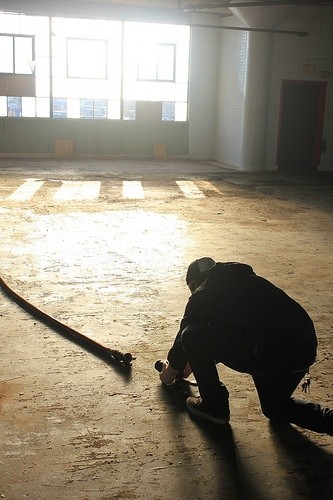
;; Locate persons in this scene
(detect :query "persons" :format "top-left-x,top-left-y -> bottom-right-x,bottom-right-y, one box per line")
159,258 -> 333,437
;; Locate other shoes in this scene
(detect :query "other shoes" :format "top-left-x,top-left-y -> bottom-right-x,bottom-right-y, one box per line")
186,396 -> 229,424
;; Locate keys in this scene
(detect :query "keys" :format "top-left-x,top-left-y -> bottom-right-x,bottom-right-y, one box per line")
302,368 -> 311,394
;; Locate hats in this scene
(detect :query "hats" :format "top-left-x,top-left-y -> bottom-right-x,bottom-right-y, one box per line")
186,257 -> 215,284
